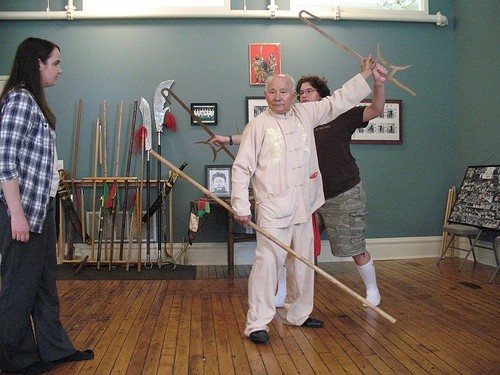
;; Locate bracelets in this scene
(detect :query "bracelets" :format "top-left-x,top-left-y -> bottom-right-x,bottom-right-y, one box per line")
229,135 -> 233,145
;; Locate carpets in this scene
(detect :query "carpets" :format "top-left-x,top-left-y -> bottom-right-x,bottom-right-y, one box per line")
55,263 -> 198,280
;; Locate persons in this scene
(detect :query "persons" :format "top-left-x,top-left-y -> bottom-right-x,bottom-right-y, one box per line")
212,62 -> 388,307
211,172 -> 228,193
231,54 -> 376,343
0,37 -> 95,375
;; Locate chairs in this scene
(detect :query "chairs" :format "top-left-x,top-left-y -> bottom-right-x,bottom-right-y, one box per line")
437,224 -> 481,272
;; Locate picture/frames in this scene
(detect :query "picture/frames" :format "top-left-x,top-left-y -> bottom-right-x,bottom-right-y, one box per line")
190,103 -> 218,126
248,43 -> 282,85
204,164 -> 233,198
350,99 -> 404,145
245,96 -> 268,124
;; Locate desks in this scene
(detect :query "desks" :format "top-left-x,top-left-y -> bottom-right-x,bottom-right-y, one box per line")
188,200 -> 257,282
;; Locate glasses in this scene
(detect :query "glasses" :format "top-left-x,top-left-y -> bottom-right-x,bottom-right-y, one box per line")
297,88 -> 319,96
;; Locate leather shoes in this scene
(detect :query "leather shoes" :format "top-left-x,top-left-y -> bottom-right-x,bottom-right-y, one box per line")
303,319 -> 323,328
251,330 -> 268,342
